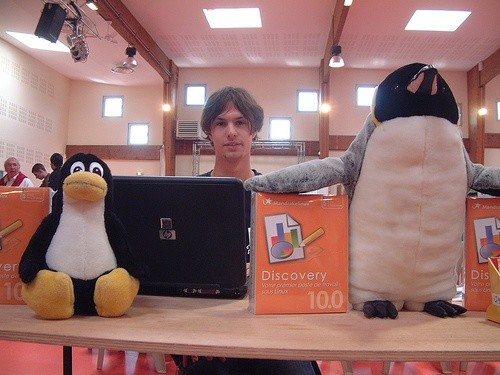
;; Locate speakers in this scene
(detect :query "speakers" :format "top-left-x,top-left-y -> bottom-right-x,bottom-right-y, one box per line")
34,3 -> 67,42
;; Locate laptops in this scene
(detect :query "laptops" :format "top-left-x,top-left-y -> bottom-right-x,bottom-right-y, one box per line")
109,176 -> 250,299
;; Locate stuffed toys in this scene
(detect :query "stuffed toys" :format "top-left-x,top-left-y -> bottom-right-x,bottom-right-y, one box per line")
242,63 -> 500,319
18,152 -> 145,320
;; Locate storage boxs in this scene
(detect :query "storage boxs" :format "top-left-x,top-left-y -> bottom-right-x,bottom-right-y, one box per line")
250,189 -> 349,315
0,186 -> 53,305
463,196 -> 500,311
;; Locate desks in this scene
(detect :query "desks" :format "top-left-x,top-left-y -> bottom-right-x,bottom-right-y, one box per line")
0,263 -> 500,375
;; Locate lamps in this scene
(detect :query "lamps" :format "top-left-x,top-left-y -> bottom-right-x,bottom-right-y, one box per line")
328,45 -> 345,68
123,42 -> 137,68
86,0 -> 99,11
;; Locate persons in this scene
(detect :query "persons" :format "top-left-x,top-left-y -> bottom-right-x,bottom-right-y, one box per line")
170,85 -> 316,375
0,153 -> 64,188
